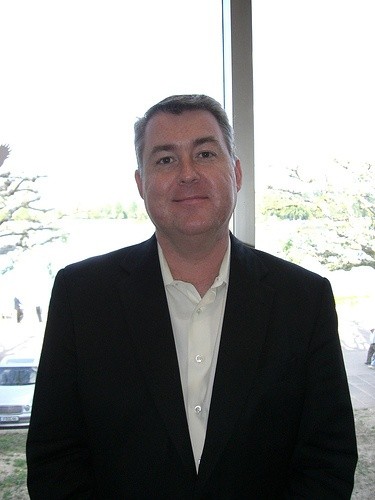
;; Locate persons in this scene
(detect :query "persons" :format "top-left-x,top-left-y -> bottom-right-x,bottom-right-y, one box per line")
25,94 -> 359,500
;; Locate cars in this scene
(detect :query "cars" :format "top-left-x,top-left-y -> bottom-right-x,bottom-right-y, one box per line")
0,354 -> 39,428
